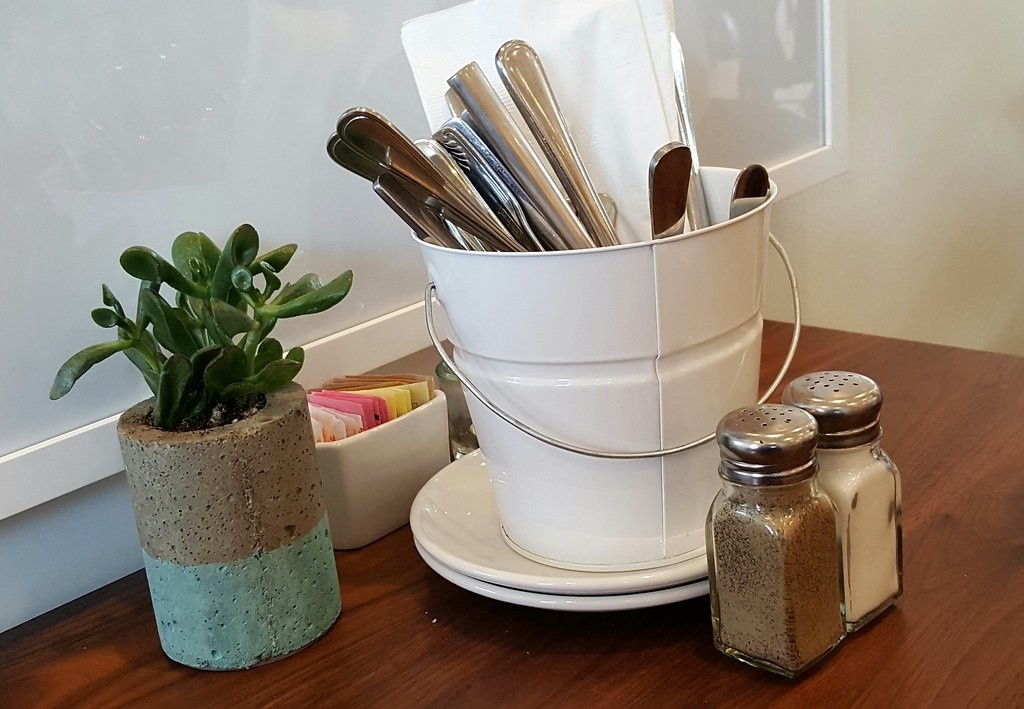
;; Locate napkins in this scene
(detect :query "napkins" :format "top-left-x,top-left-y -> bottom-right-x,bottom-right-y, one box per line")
400,0 -> 711,247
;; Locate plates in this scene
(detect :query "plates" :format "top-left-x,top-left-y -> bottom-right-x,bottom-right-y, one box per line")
411,448 -> 711,611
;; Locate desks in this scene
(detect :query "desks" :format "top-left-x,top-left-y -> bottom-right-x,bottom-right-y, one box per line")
0,317 -> 1024,709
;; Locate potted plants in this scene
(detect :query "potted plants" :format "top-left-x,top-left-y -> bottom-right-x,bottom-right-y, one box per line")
49,221 -> 356,673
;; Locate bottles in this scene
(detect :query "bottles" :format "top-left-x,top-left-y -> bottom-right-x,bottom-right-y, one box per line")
785,370 -> 904,634
707,404 -> 847,669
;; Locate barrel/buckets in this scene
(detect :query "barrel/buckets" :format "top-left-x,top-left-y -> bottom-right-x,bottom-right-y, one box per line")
413,168 -> 778,572
